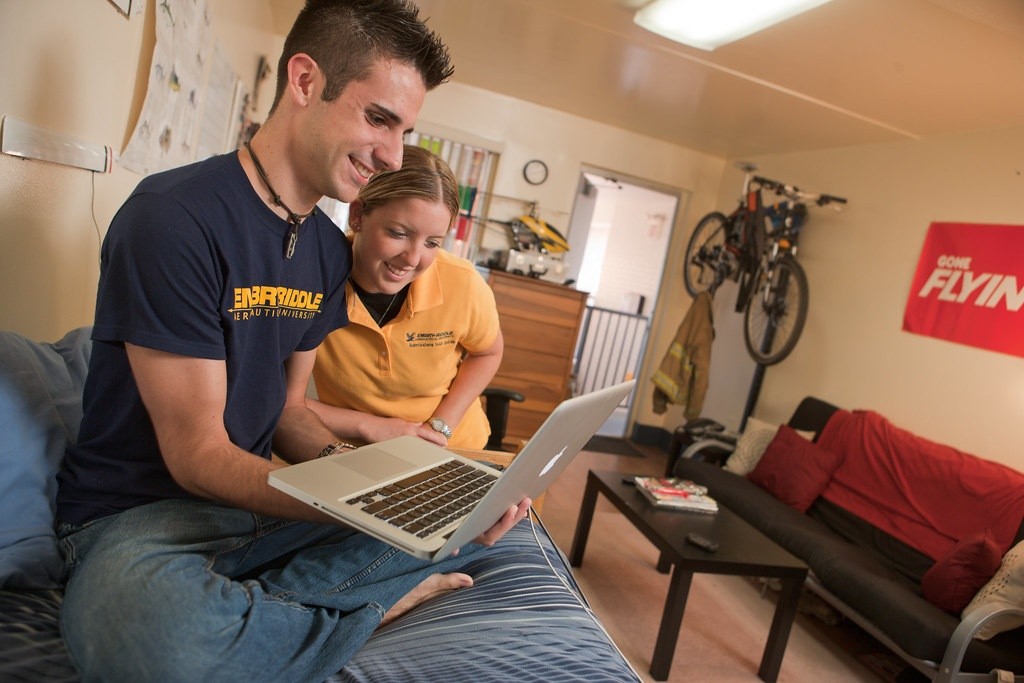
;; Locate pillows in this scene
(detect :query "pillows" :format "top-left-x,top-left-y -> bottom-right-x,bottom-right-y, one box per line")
721,416 -> 816,478
921,528 -> 1002,611
961,541 -> 1024,639
747,424 -> 843,513
1,324 -> 99,587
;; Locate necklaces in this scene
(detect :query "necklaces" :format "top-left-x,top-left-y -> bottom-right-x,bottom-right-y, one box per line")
377,291 -> 397,326
243,141 -> 315,258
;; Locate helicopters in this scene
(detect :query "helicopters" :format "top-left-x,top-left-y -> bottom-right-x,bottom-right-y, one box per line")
458,190 -> 572,261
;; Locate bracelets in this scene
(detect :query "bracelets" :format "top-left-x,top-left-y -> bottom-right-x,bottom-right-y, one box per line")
317,440 -> 356,458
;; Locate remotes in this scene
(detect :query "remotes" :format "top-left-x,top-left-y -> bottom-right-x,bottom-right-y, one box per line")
689,532 -> 720,552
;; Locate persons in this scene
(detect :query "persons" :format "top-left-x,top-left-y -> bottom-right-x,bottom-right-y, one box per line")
305,145 -> 504,450
56,0 -> 533,683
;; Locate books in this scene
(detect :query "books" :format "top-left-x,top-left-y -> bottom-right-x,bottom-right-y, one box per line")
633,477 -> 718,513
404,133 -> 483,254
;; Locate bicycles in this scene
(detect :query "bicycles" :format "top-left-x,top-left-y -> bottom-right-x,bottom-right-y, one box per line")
684,176 -> 847,365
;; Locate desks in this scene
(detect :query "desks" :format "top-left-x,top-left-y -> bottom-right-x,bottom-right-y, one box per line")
567,467 -> 810,683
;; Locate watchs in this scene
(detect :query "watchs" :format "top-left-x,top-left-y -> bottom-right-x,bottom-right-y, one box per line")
428,416 -> 452,439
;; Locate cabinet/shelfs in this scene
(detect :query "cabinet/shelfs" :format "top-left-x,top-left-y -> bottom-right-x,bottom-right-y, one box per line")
478,264 -> 590,446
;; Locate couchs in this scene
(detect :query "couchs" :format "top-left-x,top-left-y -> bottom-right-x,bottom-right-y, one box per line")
671,396 -> 1024,683
0,435 -> 645,683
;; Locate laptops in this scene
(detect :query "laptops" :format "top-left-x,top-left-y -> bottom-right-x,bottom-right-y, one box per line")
268,378 -> 637,562
